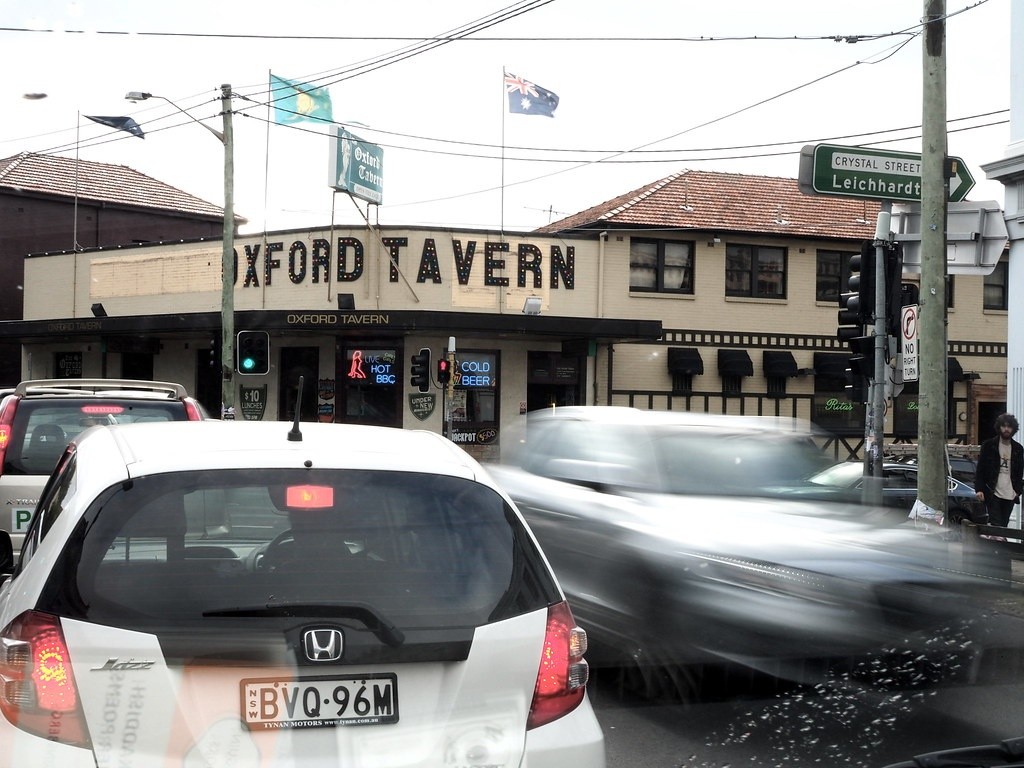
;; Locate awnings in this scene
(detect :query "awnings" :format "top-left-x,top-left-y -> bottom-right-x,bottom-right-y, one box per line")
667,347 -> 799,379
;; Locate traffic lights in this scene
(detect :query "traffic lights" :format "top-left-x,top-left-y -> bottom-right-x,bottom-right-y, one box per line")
844,368 -> 868,403
838,294 -> 863,342
411,347 -> 431,394
437,358 -> 450,384
237,329 -> 270,376
850,337 -> 874,378
848,239 -> 877,323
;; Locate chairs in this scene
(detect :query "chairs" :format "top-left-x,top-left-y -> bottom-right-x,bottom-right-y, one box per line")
98,494 -> 188,589
24,424 -> 65,474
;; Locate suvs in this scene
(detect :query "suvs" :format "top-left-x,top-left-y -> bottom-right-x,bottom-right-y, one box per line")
888,448 -> 981,492
0,376 -> 211,578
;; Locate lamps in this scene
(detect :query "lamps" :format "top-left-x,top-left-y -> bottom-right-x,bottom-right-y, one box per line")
337,293 -> 355,309
522,297 -> 543,316
708,234 -> 721,247
91,303 -> 107,316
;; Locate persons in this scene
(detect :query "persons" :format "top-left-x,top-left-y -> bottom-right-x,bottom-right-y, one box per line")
270,504 -> 350,578
975,414 -> 1024,528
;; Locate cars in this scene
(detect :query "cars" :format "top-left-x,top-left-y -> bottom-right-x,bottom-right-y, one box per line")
0,418 -> 612,768
757,460 -> 992,530
483,402 -> 1022,720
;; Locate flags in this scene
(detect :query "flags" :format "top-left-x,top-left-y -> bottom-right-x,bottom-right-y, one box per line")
504,71 -> 560,120
81,115 -> 145,140
270,73 -> 334,126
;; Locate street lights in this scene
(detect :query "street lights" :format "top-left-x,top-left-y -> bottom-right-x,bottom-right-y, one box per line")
124,93 -> 237,421
